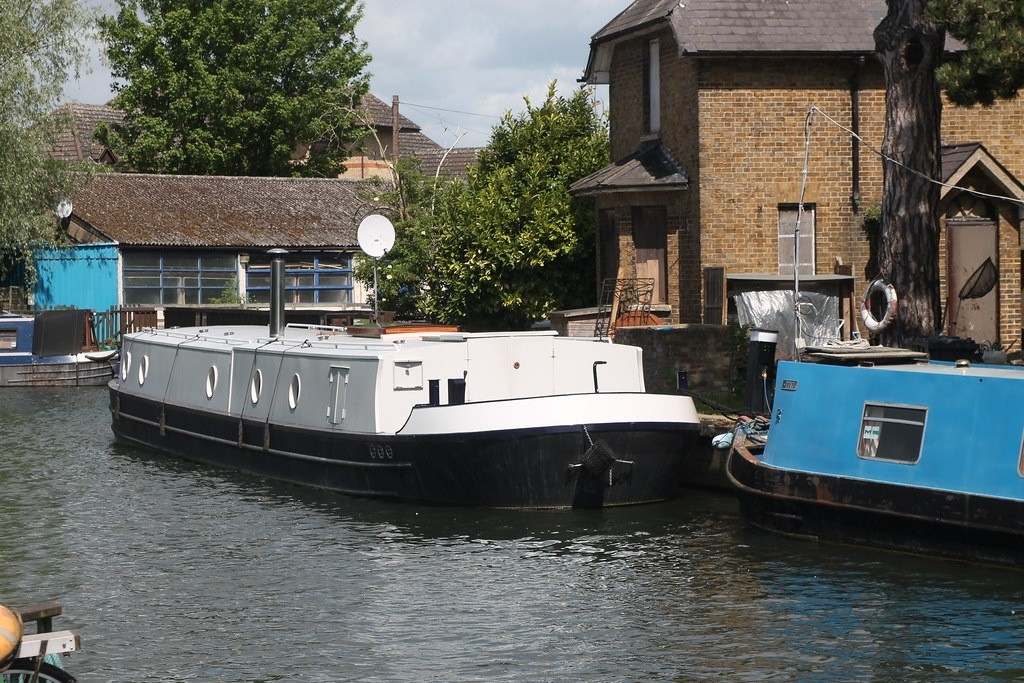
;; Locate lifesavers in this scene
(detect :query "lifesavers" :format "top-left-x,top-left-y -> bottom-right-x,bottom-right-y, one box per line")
860,276 -> 899,331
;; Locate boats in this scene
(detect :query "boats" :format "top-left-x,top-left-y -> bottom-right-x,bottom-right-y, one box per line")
107,248 -> 702,510
725,346 -> 1024,570
